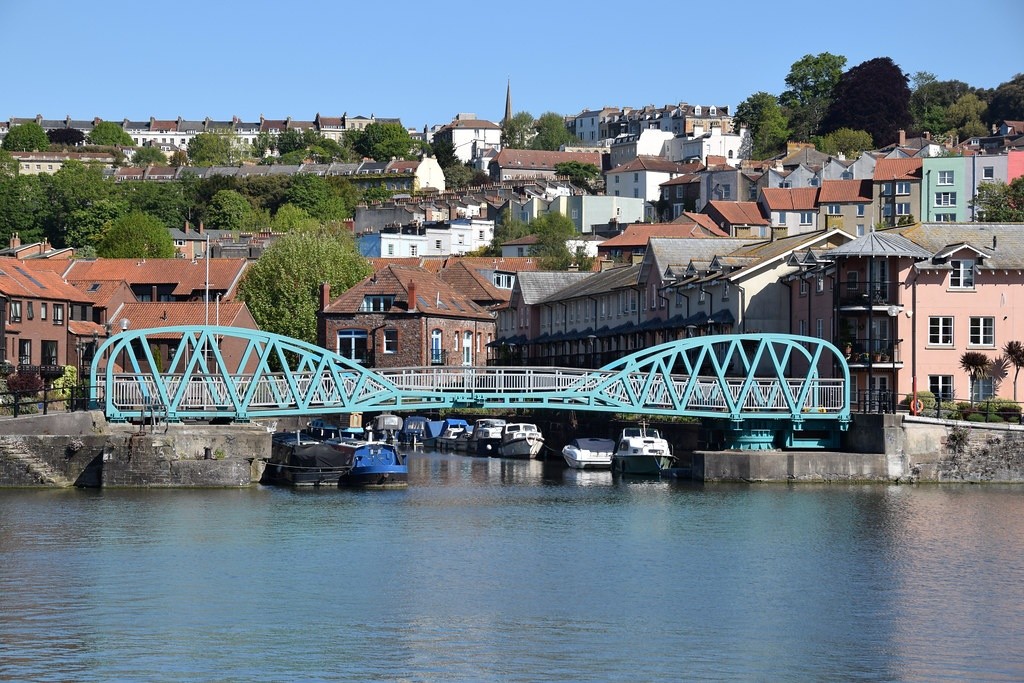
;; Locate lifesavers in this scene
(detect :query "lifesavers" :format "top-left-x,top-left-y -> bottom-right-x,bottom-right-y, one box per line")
910,398 -> 924,414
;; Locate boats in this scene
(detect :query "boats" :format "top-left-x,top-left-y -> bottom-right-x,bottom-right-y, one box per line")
263,411 -> 681,493
